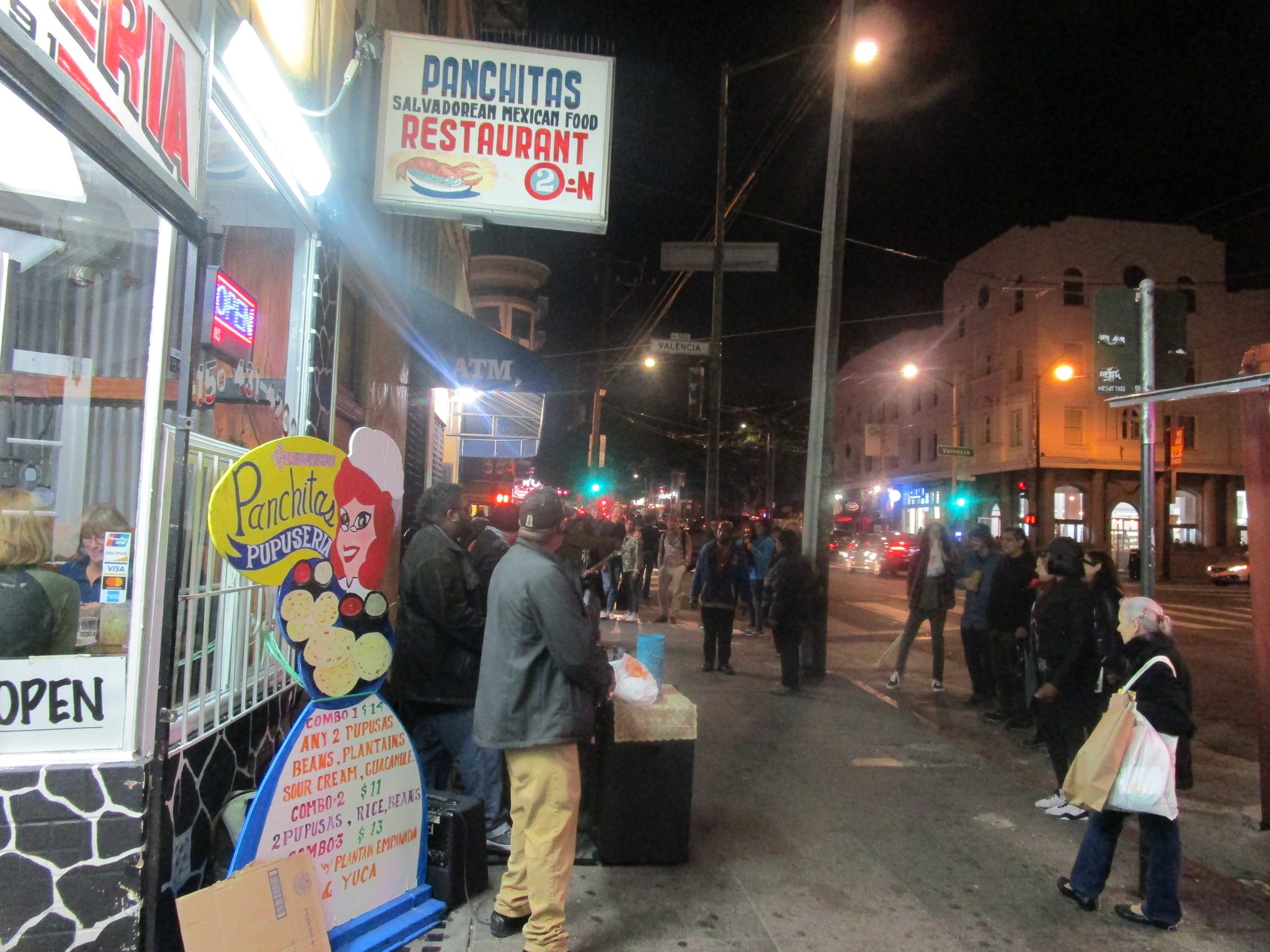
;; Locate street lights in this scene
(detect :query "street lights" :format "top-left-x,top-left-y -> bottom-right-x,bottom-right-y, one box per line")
582,355 -> 657,503
702,38 -> 884,546
1032,353 -> 1079,556
899,365 -> 959,529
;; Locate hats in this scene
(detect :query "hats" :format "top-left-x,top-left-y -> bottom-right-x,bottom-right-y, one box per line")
519,485 -> 574,529
489,502 -> 520,530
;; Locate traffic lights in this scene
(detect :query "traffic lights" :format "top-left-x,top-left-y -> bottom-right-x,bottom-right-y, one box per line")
688,366 -> 703,418
1018,473 -> 1033,501
1023,514 -> 1038,525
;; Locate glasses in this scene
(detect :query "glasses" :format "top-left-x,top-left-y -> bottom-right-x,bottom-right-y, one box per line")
453,504 -> 473,515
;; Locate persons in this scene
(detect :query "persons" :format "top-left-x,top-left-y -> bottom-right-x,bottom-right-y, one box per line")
740,517 -> 782,637
1058,598 -> 1195,931
472,489 -> 619,951
690,520 -> 753,674
762,529 -> 808,696
560,503 -> 662,622
885,522 -> 955,691
1018,537 -> 1125,820
951,524 -> 1003,708
58,502 -> 133,603
656,514 -> 692,624
398,481 -> 514,853
984,526 -> 1035,727
0,487 -> 82,658
457,504 -> 520,592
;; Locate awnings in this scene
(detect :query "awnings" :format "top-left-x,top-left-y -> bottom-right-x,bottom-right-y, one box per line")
408,288 -> 569,458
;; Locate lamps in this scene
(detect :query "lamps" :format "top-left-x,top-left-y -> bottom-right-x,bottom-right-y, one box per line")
216,17 -> 333,196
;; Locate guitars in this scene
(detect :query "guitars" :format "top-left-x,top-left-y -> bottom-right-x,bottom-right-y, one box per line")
580,550 -> 625,588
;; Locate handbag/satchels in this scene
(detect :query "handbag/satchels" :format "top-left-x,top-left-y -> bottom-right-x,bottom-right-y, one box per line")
610,653 -> 659,706
1061,692 -> 1180,822
926,538 -> 945,577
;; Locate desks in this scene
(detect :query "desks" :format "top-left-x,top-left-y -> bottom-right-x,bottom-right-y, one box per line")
585,684 -> 697,867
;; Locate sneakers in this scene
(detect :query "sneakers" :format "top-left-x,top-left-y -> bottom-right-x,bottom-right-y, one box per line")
1035,788 -> 1067,810
1044,799 -> 1089,821
487,828 -> 511,849
931,679 -> 945,693
887,671 -> 904,689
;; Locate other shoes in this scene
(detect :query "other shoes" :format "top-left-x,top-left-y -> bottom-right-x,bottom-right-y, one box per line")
605,610 -> 617,620
599,611 -> 607,618
717,661 -> 733,674
1057,874 -> 1098,912
489,911 -> 531,938
968,691 -> 1046,749
740,627 -> 765,637
618,613 -> 630,620
654,614 -> 677,624
701,659 -> 715,671
1114,904 -> 1178,931
625,616 -> 638,622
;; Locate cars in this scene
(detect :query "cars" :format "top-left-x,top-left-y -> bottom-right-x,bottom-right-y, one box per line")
1203,550 -> 1251,586
657,517 -> 705,534
826,527 -> 922,577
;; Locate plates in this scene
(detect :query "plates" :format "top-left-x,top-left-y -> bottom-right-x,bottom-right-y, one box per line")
0,462 -> 54,508
75,636 -> 97,649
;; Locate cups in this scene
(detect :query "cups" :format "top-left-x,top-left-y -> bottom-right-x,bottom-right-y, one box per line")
970,570 -> 983,587
77,606 -> 99,639
99,599 -> 131,645
636,634 -> 665,696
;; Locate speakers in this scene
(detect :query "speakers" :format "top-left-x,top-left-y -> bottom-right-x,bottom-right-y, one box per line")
424,789 -> 488,909
